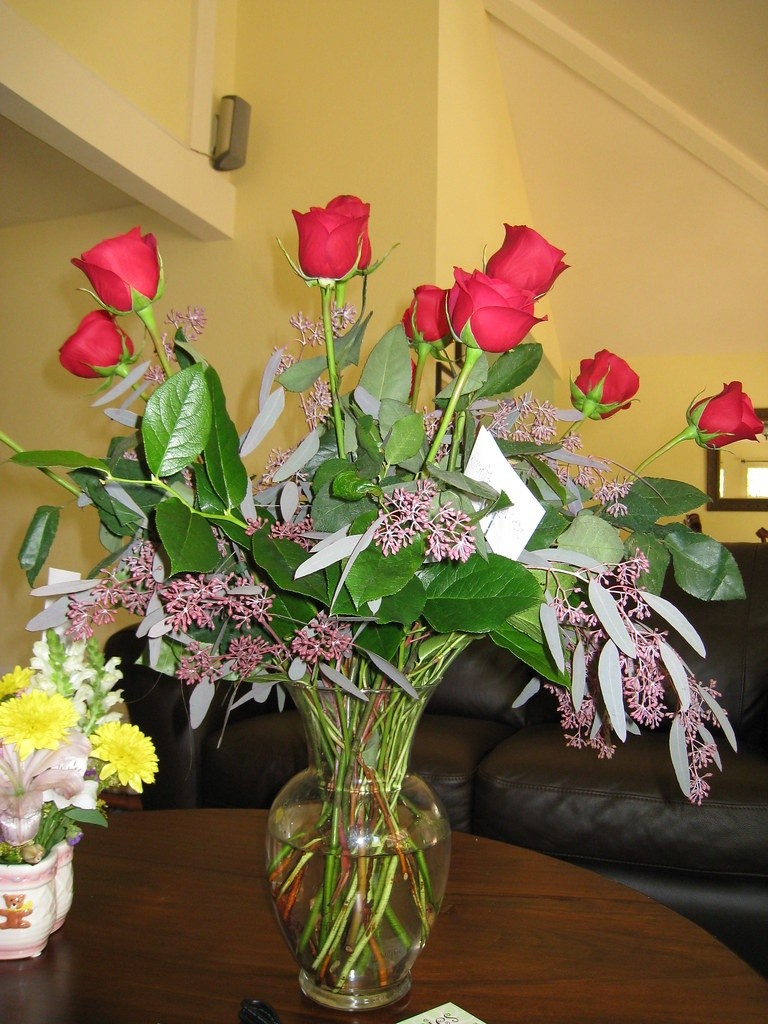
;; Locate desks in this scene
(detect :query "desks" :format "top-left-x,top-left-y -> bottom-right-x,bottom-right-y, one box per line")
1,810 -> 768,1024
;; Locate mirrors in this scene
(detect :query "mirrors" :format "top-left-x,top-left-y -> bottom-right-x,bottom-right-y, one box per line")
706,407 -> 768,510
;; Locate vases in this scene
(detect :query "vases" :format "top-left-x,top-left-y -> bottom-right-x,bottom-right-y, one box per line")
259,679 -> 452,1011
0,839 -> 73,965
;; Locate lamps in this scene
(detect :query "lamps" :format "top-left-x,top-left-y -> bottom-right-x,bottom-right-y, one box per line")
212,94 -> 252,172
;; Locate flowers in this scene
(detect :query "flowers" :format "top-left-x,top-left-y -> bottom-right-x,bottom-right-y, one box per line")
2,194 -> 767,986
1,615 -> 161,868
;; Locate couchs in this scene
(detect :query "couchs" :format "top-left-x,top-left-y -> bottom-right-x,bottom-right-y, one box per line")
103,540 -> 766,980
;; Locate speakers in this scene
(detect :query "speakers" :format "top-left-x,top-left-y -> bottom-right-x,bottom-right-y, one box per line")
212,95 -> 252,171
